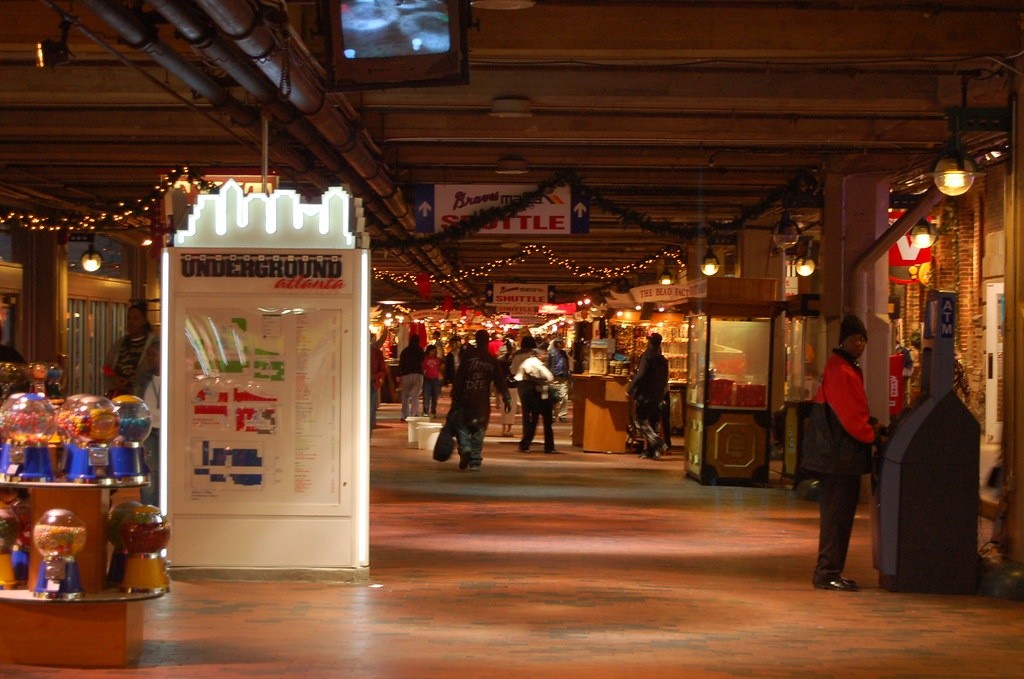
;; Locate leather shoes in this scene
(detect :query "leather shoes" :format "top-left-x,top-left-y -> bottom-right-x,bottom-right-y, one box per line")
812,574 -> 858,590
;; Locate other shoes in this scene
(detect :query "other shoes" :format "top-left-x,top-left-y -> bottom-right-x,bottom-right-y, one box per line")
544,448 -> 559,454
458,448 -> 472,469
655,443 -> 668,456
519,447 -> 529,452
503,431 -> 514,437
470,463 -> 481,470
552,418 -> 556,422
560,418 -> 567,422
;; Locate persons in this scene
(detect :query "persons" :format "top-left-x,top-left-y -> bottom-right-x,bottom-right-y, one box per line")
102,303 -> 163,515
0,320 -> 25,363
450,328 -> 511,470
370,326 -> 475,430
896,336 -> 914,379
801,316 -> 885,590
625,332 -> 669,458
489,332 -> 574,455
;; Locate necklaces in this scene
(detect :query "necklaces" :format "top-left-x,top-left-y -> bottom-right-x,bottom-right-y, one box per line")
152,380 -> 160,409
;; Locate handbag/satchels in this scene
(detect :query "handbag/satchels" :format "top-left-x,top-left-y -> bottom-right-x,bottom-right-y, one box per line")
432,410 -> 454,461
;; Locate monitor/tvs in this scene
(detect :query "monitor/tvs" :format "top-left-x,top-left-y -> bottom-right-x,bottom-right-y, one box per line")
329,0 -> 463,81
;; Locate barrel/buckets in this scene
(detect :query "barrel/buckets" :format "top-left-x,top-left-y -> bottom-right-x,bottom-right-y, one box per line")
405,416 -> 431,444
415,422 -> 444,451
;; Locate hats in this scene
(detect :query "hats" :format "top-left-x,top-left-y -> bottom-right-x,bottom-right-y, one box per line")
838,313 -> 868,345
646,332 -> 663,344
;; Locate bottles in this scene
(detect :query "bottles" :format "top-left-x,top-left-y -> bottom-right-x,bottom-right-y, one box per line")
609,360 -> 632,375
